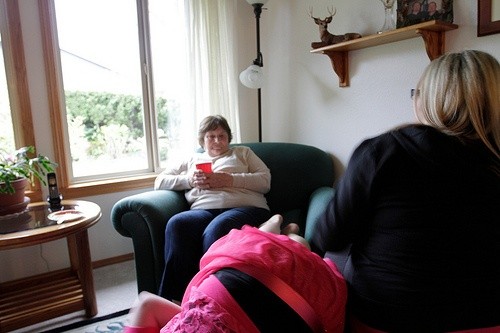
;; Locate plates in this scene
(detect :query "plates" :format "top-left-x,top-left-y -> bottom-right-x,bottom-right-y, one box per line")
47,209 -> 84,223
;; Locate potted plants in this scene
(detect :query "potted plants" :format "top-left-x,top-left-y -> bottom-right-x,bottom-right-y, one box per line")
0,146 -> 59,206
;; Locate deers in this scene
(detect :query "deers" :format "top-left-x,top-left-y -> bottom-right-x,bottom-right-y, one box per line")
305,3 -> 363,44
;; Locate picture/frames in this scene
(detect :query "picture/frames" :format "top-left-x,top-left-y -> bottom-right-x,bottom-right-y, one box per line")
396,0 -> 453,28
477,0 -> 500,37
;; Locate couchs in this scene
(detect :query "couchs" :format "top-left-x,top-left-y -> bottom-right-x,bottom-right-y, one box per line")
110,143 -> 337,300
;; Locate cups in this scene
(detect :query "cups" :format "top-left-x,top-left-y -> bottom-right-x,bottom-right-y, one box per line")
195,162 -> 212,179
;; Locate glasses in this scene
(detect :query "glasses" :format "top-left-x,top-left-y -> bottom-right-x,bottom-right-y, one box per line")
204,133 -> 227,142
410,87 -> 421,100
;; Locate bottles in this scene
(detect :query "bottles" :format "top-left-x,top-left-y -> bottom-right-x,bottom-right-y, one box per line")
376,0 -> 396,35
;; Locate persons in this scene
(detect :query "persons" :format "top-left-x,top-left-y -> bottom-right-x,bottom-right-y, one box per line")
310,49 -> 500,333
153,114 -> 271,308
119,214 -> 345,333
405,2 -> 442,26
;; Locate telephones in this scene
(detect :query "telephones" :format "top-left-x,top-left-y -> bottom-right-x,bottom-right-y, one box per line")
47,173 -> 64,212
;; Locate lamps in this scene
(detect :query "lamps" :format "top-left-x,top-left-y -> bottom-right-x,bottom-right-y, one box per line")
239,0 -> 267,143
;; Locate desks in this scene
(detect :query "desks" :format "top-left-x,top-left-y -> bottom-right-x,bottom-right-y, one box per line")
0,199 -> 103,333
